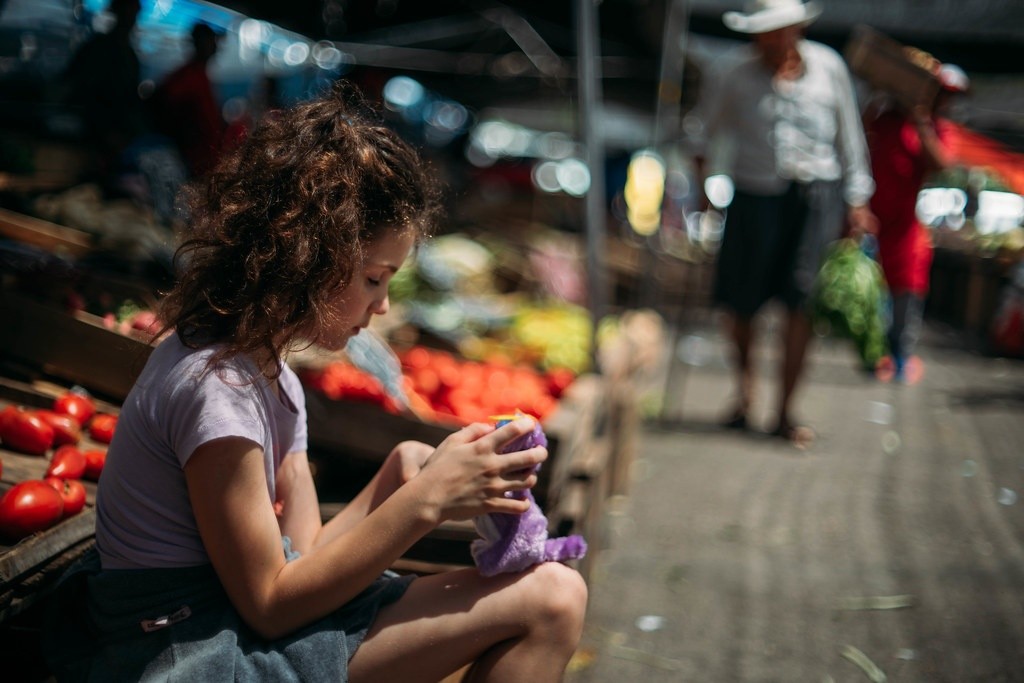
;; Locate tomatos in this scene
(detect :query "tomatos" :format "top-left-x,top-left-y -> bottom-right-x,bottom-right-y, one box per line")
1,392 -> 120,532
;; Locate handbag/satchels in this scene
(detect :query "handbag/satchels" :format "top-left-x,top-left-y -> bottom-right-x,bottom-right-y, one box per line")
808,231 -> 895,370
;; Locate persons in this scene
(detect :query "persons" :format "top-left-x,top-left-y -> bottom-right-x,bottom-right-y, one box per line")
1,0 -> 289,179
83,1 -> 590,683
842,39 -> 966,386
699,0 -> 881,445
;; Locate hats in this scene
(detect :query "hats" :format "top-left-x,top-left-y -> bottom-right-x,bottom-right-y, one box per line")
717,0 -> 823,38
182,19 -> 227,42
